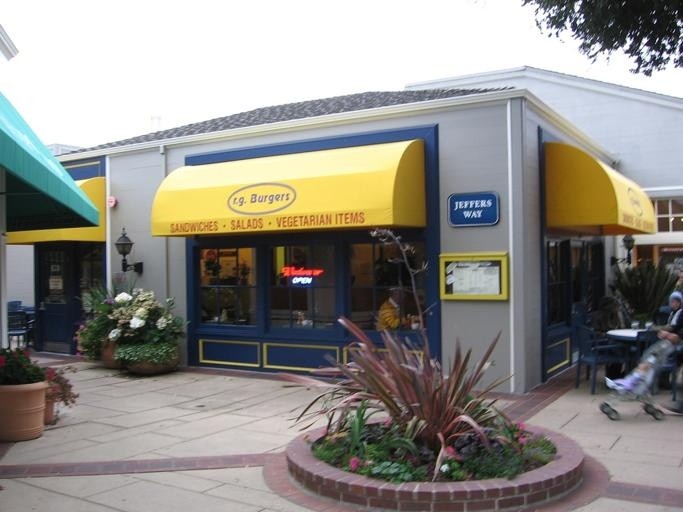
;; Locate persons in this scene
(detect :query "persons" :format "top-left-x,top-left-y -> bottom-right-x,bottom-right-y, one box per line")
648,290 -> 682,391
602,321 -> 683,401
570,301 -> 622,388
374,286 -> 419,330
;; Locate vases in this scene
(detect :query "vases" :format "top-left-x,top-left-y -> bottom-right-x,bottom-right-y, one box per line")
1,379 -> 50,442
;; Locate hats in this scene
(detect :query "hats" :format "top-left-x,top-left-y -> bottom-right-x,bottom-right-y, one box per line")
669,292 -> 682,304
388,282 -> 413,294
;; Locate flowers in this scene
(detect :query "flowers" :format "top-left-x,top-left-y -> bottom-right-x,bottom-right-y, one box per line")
0,346 -> 79,424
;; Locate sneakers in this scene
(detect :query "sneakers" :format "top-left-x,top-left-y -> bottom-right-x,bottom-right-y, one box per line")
661,399 -> 683,414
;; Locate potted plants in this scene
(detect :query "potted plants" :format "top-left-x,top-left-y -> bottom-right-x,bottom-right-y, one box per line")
75,275 -> 191,375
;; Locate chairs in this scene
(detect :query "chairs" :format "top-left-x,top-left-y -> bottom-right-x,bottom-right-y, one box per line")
574,326 -> 660,396
8,309 -> 37,349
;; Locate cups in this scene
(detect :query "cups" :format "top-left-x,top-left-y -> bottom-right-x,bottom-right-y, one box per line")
630,320 -> 639,336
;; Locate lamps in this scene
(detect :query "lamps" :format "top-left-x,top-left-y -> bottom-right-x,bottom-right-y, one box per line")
610,232 -> 635,268
114,227 -> 143,273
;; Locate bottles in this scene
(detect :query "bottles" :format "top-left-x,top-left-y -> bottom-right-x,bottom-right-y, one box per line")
297,312 -> 304,327
